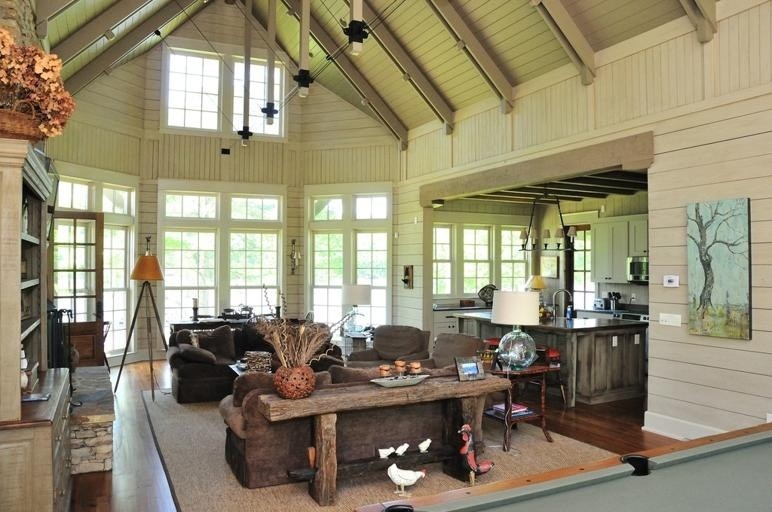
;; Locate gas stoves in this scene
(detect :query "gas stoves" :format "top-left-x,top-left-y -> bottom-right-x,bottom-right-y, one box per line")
615,310 -> 649,316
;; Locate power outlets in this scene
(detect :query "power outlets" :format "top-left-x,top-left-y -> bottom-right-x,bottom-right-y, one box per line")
660,313 -> 682,327
631,293 -> 636,300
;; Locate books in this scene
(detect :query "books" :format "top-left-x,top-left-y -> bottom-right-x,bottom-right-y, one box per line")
25,360 -> 39,372
20,392 -> 51,402
492,401 -> 533,418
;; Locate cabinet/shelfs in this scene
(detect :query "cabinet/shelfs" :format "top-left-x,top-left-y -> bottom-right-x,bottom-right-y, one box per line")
434,312 -> 460,335
591,222 -> 629,283
629,220 -> 649,257
0,368 -> 71,512
1,139 -> 47,419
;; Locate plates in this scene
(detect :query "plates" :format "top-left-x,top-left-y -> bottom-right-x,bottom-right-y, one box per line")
370,375 -> 431,388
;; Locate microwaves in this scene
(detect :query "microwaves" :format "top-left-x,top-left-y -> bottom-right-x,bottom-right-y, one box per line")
626,257 -> 649,282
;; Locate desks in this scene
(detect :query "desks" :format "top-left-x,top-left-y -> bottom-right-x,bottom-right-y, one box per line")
258,374 -> 511,505
170,319 -> 256,332
487,367 -> 562,453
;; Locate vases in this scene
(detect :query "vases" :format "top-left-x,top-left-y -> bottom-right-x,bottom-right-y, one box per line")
274,365 -> 316,399
1,101 -> 43,141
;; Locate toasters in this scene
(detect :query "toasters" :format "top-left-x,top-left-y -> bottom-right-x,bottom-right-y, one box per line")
593,298 -> 610,310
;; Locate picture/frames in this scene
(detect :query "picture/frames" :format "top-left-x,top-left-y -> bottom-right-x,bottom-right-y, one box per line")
454,356 -> 486,382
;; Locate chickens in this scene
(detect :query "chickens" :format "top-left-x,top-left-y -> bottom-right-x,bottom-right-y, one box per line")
388,463 -> 427,497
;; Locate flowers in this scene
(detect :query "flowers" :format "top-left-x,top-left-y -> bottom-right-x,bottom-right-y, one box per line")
251,283 -> 365,367
0,29 -> 75,138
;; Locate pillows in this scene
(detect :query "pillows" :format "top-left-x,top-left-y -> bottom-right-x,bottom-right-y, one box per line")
329,364 -> 394,384
232,372 -> 331,408
211,326 -> 234,358
179,344 -> 214,362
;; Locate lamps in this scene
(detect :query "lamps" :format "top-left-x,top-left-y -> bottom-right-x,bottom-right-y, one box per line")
543,229 -> 550,250
567,226 -> 577,248
289,239 -> 303,266
112,255 -> 166,402
530,229 -> 539,249
519,229 -> 528,250
145,235 -> 153,256
491,291 -> 539,370
555,229 -> 563,248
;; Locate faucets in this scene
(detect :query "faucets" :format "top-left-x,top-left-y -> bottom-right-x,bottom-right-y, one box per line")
552,289 -> 572,319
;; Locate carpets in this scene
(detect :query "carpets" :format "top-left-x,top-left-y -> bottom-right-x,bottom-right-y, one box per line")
140,389 -> 624,511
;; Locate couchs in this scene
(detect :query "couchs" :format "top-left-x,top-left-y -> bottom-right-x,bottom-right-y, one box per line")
220,366 -> 486,491
407,332 -> 489,369
346,325 -> 430,369
166,326 -> 344,403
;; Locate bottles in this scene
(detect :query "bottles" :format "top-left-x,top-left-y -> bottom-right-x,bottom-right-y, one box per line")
410,362 -> 422,379
394,361 -> 406,378
379,365 -> 391,380
